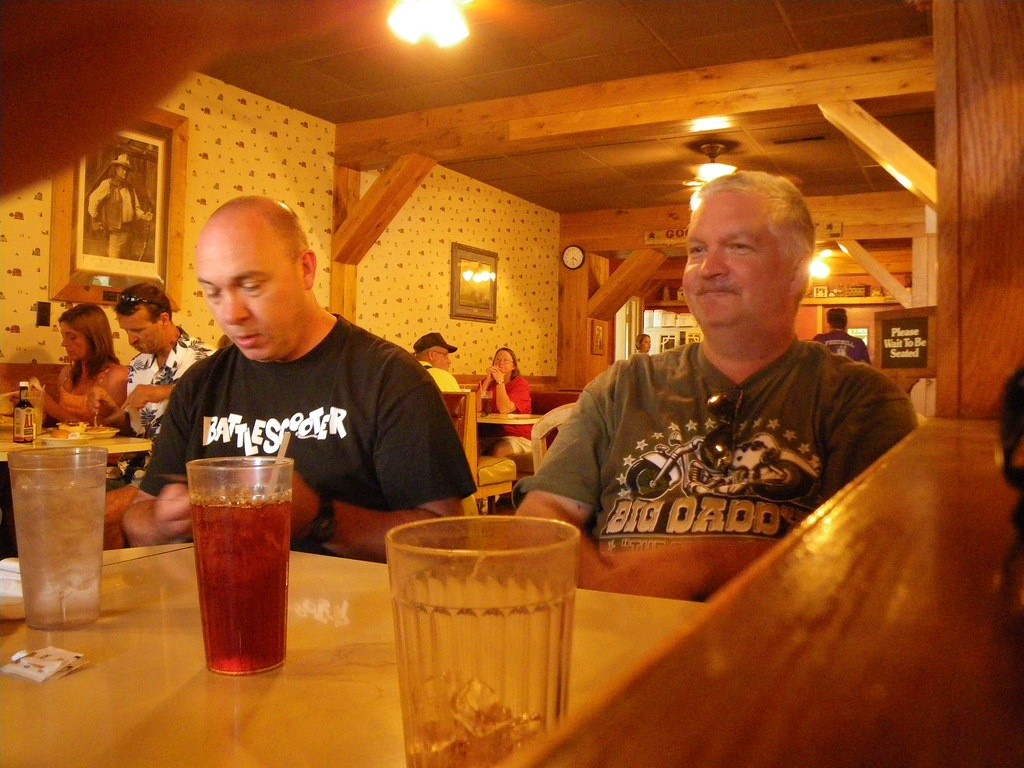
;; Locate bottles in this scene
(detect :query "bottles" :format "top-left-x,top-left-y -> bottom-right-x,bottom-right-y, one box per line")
13,381 -> 35,443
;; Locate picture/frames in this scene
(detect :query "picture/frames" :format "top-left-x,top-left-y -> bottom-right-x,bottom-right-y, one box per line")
590,319 -> 605,354
450,241 -> 499,323
49,104 -> 190,311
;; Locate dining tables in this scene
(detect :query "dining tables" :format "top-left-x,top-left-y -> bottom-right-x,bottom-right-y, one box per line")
0,536 -> 713,768
0,424 -> 153,466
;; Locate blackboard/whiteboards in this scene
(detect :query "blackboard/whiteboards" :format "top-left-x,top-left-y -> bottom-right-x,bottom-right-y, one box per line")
875,306 -> 938,378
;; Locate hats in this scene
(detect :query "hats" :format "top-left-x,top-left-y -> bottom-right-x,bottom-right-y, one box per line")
411,332 -> 458,355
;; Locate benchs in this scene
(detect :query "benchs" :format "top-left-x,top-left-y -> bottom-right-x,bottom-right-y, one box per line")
439,386 -> 582,515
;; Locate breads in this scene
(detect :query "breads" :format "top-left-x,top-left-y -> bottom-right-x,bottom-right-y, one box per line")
50,429 -> 71,439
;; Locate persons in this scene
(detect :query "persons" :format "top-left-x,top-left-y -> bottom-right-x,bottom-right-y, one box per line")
408,332 -> 462,393
510,169 -> 919,603
472,347 -> 535,458
87,281 -> 216,549
120,193 -> 478,564
811,309 -> 872,366
25,302 -> 131,430
87,154 -> 155,263
634,333 -> 652,355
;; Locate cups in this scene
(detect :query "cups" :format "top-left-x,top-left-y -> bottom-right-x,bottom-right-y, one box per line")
186,457 -> 295,677
480,390 -> 493,416
386,515 -> 579,768
27,390 -> 44,435
7,445 -> 109,631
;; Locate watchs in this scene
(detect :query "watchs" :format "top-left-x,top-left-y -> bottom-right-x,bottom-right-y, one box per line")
308,489 -> 337,546
495,381 -> 504,385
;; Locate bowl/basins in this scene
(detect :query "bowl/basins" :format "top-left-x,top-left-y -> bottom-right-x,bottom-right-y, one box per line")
56,423 -> 89,433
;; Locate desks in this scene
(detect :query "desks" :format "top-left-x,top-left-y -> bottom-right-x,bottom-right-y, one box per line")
477,413 -> 544,425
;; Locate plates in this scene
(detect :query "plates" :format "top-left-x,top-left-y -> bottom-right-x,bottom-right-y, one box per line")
79,426 -> 121,438
38,435 -> 94,447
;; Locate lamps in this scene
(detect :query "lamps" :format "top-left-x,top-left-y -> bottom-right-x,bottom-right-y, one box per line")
689,182 -> 722,212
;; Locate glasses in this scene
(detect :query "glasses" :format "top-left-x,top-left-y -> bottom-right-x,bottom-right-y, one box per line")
493,358 -> 515,364
431,349 -> 449,358
699,388 -> 744,478
114,292 -> 169,310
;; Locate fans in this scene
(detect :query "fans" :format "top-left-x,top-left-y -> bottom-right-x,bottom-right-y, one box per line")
642,140 -> 803,201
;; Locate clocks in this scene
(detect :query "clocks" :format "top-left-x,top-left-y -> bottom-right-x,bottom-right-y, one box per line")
562,244 -> 584,270
